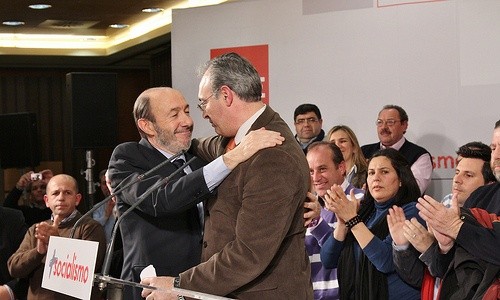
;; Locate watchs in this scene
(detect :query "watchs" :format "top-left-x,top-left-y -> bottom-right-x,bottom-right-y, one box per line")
173,276 -> 183,300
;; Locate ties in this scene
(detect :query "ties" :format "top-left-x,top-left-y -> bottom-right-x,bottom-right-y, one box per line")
224,137 -> 235,154
172,159 -> 187,177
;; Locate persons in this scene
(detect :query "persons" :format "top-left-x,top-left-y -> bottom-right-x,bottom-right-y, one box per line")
0,170 -> 125,300
105,51 -> 316,300
108,87 -> 322,299
291,101 -> 500,300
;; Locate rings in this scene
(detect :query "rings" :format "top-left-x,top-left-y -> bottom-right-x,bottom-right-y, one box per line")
412,234 -> 416,239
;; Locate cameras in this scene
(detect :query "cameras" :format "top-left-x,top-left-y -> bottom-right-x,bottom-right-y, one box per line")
31,173 -> 42,180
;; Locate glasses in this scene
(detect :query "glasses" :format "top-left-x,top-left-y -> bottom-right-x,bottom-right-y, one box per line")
294,117 -> 320,124
376,119 -> 402,126
198,88 -> 219,111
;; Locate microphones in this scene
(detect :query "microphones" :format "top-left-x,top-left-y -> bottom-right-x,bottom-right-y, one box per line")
104,156 -> 197,278
69,150 -> 185,238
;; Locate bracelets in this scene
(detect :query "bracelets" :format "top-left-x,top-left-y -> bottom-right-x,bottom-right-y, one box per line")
344,215 -> 365,229
